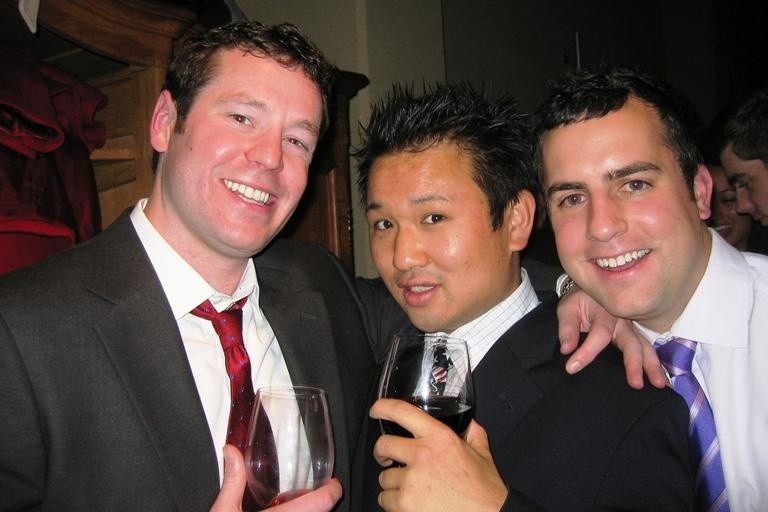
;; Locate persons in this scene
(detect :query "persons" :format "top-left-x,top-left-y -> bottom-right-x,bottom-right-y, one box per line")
700,139 -> 753,251
361,76 -> 692,512
719,87 -> 768,229
0,21 -> 671,512
532,63 -> 768,512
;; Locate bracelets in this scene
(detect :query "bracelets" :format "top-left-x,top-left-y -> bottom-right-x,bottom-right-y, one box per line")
560,280 -> 574,299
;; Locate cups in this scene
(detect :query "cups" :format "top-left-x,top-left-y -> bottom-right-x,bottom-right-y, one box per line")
378,333 -> 475,443
245,385 -> 336,510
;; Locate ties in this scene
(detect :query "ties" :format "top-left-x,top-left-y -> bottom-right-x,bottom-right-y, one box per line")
427,339 -> 453,398
650,338 -> 730,512
189,296 -> 279,511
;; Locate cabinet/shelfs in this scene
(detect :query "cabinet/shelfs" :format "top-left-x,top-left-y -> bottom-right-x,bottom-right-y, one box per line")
0,0 -> 369,285
